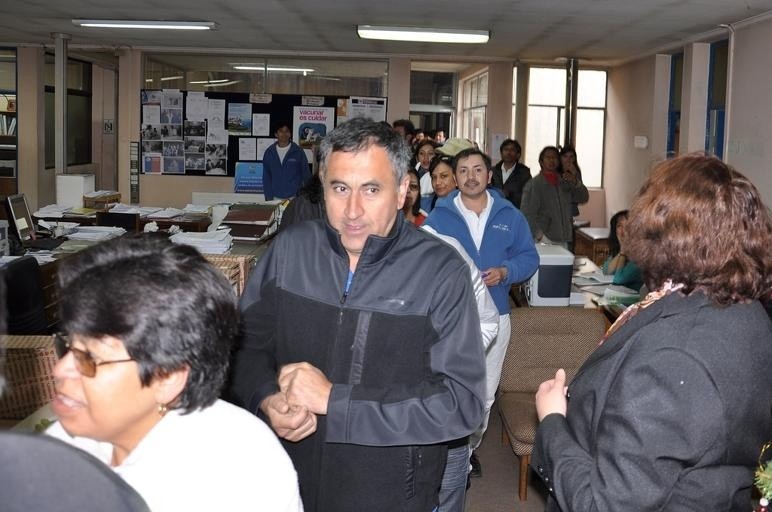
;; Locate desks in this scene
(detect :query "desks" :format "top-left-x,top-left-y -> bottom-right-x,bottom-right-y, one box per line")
31,204 -> 280,238
508,252 -> 641,310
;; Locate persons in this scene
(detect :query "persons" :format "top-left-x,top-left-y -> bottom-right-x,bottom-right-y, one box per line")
262,120 -> 651,294
419,148 -> 540,489
418,225 -> 500,512
530,151 -> 772,512
42,232 -> 305,512
225,116 -> 486,512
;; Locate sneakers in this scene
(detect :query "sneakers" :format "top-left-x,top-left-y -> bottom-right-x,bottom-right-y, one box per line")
466,452 -> 481,491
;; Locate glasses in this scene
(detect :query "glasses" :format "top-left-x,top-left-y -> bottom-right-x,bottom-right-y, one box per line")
53,332 -> 133,378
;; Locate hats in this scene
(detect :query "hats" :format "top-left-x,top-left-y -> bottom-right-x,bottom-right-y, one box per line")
435,137 -> 473,156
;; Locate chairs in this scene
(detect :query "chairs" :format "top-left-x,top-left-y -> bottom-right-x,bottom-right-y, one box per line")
496,306 -> 612,502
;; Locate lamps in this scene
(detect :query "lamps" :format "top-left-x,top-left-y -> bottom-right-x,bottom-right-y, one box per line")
352,24 -> 495,44
234,65 -> 315,73
71,17 -> 220,32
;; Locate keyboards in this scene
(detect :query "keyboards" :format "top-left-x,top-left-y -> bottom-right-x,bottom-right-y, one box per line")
29,236 -> 63,251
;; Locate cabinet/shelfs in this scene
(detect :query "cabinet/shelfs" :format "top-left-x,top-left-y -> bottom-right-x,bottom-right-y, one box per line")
574,226 -> 611,266
0,111 -> 17,202
0,221 -> 128,334
84,191 -> 122,209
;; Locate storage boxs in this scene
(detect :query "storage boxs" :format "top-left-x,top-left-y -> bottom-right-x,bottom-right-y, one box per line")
56,173 -> 95,208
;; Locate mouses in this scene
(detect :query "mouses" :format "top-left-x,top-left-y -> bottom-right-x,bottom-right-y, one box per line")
57,234 -> 67,240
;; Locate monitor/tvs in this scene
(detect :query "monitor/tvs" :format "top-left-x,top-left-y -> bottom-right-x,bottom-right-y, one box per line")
5,192 -> 37,248
